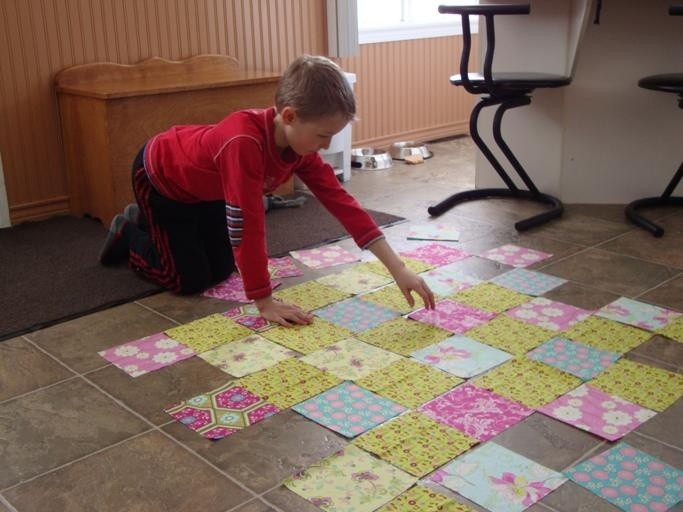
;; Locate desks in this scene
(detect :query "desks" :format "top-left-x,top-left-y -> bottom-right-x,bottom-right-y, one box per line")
54,55 -> 294,230
296,71 -> 357,193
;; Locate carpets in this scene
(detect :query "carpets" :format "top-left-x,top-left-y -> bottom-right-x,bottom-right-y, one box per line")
1,192 -> 410,342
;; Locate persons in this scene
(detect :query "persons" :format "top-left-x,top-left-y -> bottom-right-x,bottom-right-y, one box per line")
96,54 -> 437,327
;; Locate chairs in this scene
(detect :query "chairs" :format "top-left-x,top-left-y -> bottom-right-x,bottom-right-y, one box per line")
428,5 -> 571,233
625,6 -> 683,239
